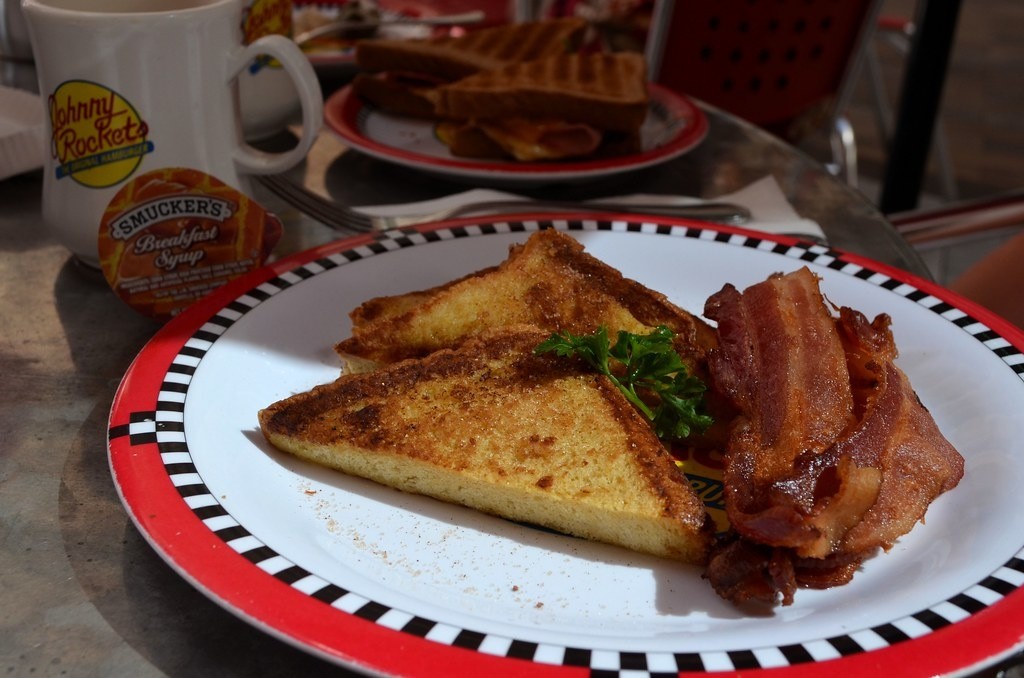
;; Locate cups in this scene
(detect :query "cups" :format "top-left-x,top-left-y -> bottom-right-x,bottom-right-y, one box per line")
21,0 -> 324,273
231,0 -> 299,142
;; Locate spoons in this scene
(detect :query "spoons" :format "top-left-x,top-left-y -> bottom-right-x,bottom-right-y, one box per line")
294,12 -> 484,45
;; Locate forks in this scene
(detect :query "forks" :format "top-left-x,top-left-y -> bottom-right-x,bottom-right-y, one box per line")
260,172 -> 751,238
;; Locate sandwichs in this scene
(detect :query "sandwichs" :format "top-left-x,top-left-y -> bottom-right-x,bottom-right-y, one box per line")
353,14 -> 651,160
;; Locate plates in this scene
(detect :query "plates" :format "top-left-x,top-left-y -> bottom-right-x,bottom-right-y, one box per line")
322,78 -> 710,184
99,203 -> 1024,678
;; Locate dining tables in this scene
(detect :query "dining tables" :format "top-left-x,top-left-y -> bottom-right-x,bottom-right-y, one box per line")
0,88 -> 1023,678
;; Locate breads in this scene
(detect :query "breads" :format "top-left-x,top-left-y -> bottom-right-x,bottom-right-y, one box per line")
256,224 -> 729,568
